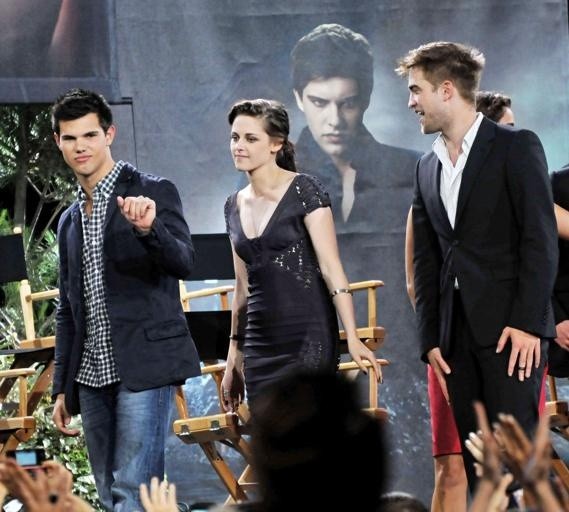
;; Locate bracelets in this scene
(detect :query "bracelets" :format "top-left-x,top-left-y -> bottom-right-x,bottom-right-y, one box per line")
228,334 -> 245,342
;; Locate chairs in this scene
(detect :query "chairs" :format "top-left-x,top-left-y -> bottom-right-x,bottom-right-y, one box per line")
545,375 -> 569,439
172,279 -> 389,512
0,277 -> 76,459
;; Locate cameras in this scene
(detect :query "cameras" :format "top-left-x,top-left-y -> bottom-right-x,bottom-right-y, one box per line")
6,448 -> 46,470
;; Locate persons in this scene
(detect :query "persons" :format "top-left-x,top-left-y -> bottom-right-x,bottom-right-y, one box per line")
550,163 -> 569,388
405,86 -> 569,512
392,39 -> 562,505
1,364 -> 569,510
282,22 -> 440,509
48,86 -> 201,512
218,99 -> 384,412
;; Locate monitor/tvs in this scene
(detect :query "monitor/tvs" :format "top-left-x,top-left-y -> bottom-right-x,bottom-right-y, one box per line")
0,0 -> 124,106
